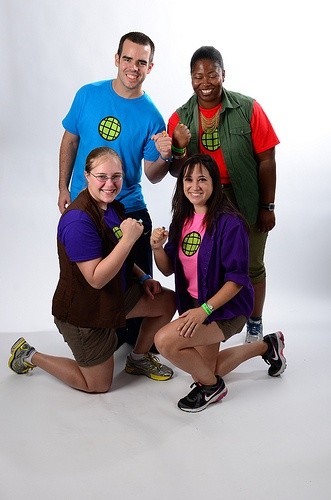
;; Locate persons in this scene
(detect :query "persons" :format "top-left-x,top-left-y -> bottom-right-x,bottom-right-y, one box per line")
8,147 -> 177,393
150,154 -> 287,413
168,46 -> 280,344
58,32 -> 172,353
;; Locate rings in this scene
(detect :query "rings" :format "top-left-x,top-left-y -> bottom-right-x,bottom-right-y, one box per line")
162,131 -> 167,137
178,125 -> 182,128
137,219 -> 143,226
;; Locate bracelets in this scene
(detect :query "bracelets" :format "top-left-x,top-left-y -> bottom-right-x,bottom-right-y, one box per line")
201,303 -> 212,315
173,152 -> 186,159
159,156 -> 171,162
172,145 -> 185,153
141,275 -> 152,284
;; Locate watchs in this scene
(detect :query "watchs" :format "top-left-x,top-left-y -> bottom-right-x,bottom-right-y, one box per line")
207,303 -> 214,311
261,203 -> 275,211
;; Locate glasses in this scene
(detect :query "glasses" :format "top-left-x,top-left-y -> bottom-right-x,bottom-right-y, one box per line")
88,171 -> 125,182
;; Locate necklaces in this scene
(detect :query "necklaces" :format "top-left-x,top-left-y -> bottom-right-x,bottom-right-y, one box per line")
199,108 -> 220,134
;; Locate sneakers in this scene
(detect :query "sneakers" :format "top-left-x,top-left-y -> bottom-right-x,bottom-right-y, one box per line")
262,330 -> 287,378
178,375 -> 227,413
124,352 -> 174,380
8,336 -> 37,374
243,316 -> 264,343
